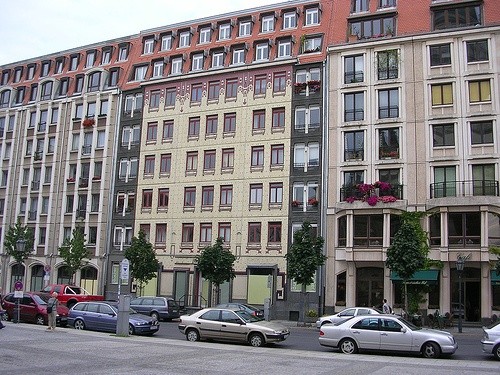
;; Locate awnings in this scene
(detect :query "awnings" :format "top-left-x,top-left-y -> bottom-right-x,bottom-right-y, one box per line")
491,271 -> 500,285
389,269 -> 439,284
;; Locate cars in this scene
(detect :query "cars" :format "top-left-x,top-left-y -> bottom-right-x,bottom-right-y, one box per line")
177,307 -> 290,347
216,303 -> 266,321
480,323 -> 500,361
316,307 -> 387,329
318,313 -> 459,359
0,292 -> 69,325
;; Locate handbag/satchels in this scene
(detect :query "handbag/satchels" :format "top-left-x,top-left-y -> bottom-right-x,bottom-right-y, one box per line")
388,305 -> 392,314
47,307 -> 52,313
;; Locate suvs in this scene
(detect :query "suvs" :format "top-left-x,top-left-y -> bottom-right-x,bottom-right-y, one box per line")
67,301 -> 160,335
129,295 -> 182,321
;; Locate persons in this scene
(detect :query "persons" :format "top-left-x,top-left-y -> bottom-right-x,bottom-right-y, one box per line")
382,299 -> 392,314
47,292 -> 59,330
0,287 -> 7,329
405,309 -> 453,327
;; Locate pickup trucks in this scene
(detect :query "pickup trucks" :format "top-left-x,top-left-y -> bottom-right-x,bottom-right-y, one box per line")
41,283 -> 105,310
